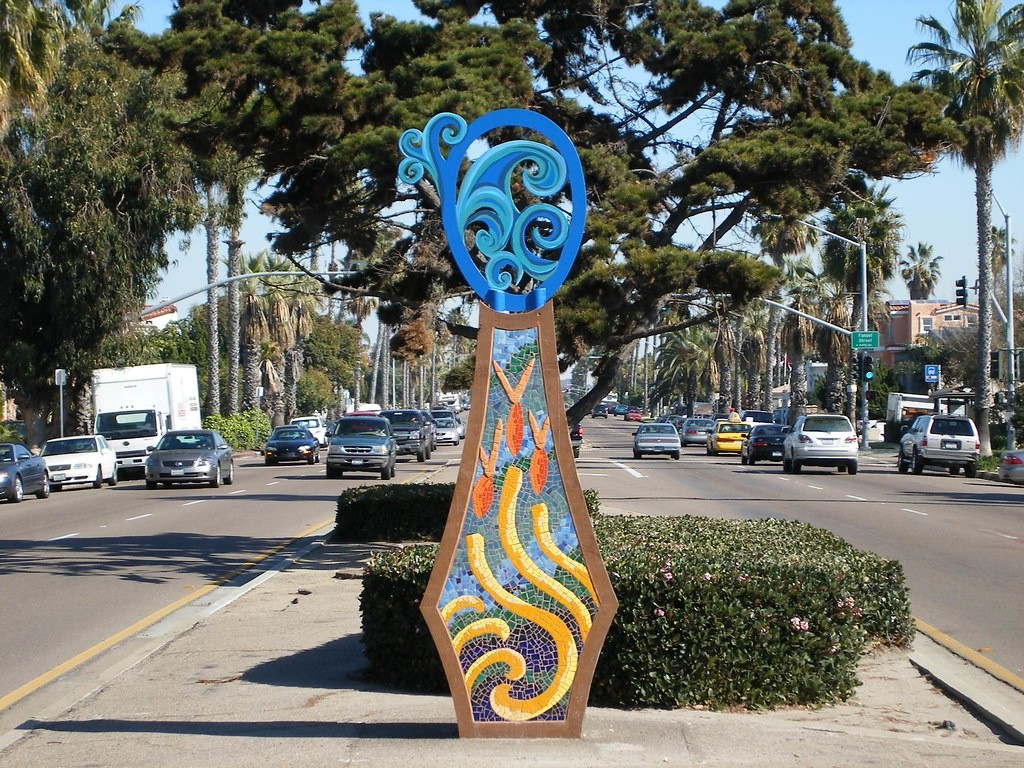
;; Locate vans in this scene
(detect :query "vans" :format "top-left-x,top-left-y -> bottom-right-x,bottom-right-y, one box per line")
738,409 -> 776,427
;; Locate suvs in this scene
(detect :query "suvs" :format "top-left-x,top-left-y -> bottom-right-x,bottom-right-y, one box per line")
421,409 -> 437,451
380,409 -> 431,462
710,412 -> 733,421
898,415 -> 980,478
325,416 -> 399,481
345,411 -> 378,431
780,413 -> 858,475
591,405 -> 608,419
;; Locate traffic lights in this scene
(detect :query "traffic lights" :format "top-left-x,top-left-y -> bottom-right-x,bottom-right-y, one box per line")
990,350 -> 1008,380
861,353 -> 875,382
661,305 -> 668,312
866,390 -> 877,400
853,354 -> 862,380
955,275 -> 967,309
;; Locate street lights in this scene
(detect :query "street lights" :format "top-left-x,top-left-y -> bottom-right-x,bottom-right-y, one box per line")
769,212 -> 871,451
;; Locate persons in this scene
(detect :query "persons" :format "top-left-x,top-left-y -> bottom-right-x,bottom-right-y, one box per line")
646,427 -> 650,431
409,416 -> 419,422
444,413 -> 448,417
690,421 -> 696,426
197,440 -> 210,450
301,434 -> 305,439
757,429 -> 766,435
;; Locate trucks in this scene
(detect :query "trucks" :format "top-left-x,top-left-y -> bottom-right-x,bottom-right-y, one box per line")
82,363 -> 203,479
885,391 -> 976,435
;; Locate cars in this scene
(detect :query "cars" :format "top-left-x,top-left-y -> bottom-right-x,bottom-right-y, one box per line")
0,442 -> 50,503
433,417 -> 460,446
998,448 -> 1024,484
632,422 -> 682,460
272,425 -> 307,440
569,423 -> 583,458
263,428 -> 321,466
678,418 -> 716,447
740,424 -> 789,465
144,430 -> 235,489
289,417 -> 330,448
601,401 -> 642,422
652,415 -> 688,432
431,392 -> 471,439
707,412 -> 752,456
38,434 -> 118,492
